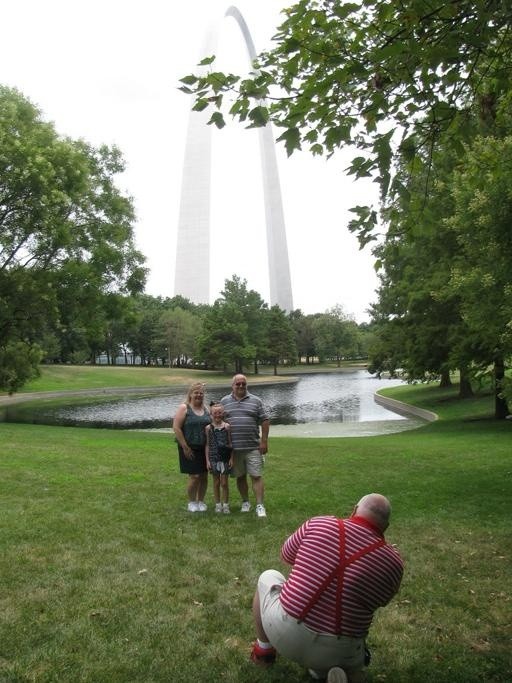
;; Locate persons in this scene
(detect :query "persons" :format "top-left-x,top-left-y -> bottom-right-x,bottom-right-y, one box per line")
208,396 -> 224,421
173,381 -> 213,513
204,402 -> 234,515
218,374 -> 270,518
249,492 -> 404,683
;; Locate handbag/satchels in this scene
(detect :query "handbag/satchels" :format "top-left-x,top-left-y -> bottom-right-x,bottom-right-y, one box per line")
217,447 -> 233,461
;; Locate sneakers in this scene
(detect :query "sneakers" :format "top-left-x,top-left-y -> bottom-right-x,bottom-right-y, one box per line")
187,502 -> 208,512
250,640 -> 276,665
241,502 -> 251,512
327,667 -> 348,683
256,504 -> 266,517
215,503 -> 230,514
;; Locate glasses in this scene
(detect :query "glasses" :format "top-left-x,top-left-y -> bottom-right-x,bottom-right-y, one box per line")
236,383 -> 247,386
